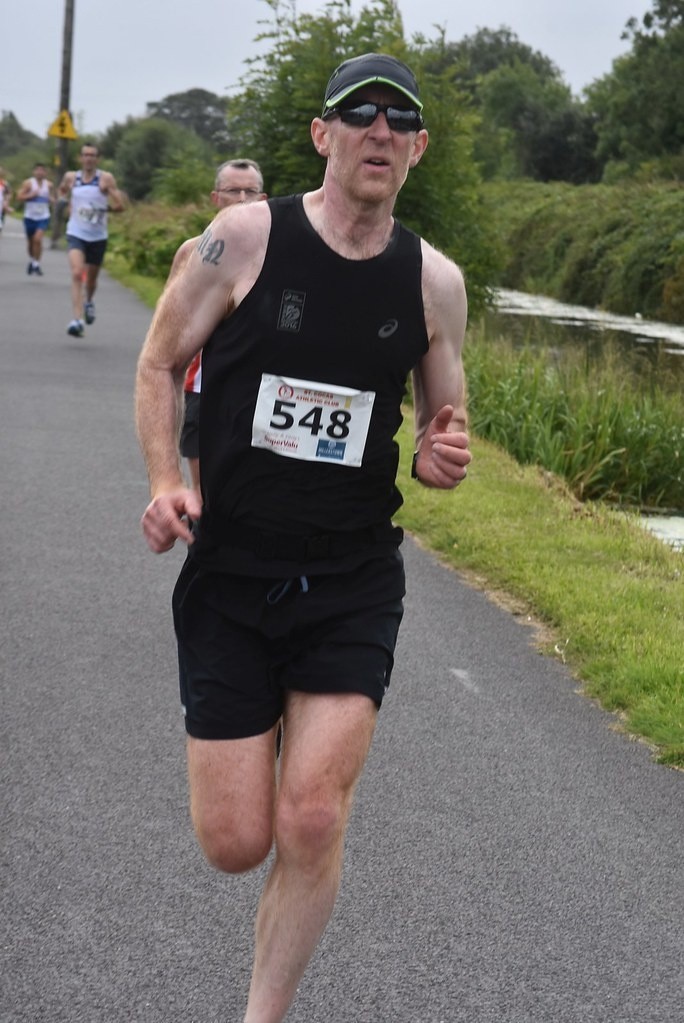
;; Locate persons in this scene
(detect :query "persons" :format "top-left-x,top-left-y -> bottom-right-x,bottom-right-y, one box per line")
0,164 -> 56,276
58,142 -> 125,337
142,53 -> 471,1023
166,161 -> 266,494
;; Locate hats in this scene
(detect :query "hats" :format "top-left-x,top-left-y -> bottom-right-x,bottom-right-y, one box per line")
322,53 -> 423,112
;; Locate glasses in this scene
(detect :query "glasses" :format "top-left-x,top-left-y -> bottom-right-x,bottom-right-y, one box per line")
217,188 -> 262,198
322,101 -> 423,132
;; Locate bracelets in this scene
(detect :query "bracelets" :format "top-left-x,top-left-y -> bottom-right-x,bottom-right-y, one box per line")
411,450 -> 420,481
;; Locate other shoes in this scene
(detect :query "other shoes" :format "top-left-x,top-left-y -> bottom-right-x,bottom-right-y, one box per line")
28,263 -> 42,276
85,303 -> 95,324
67,319 -> 86,337
